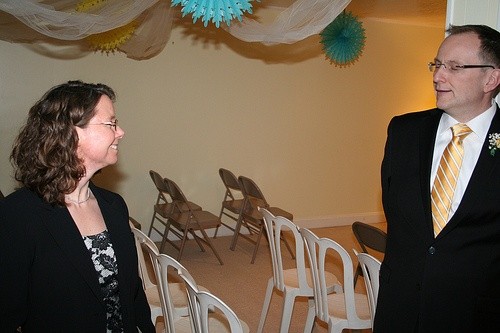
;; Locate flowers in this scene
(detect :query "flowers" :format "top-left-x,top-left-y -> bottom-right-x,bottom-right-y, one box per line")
488,133 -> 500,156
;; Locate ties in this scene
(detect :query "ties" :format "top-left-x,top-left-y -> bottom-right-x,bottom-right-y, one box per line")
431,123 -> 475,241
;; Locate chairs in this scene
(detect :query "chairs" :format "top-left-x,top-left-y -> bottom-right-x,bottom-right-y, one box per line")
128,168 -> 389,333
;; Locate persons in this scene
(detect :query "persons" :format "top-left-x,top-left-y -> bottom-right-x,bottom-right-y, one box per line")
373,23 -> 500,333
0,80 -> 156,333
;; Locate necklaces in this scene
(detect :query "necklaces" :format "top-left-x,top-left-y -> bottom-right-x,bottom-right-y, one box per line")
65,195 -> 90,205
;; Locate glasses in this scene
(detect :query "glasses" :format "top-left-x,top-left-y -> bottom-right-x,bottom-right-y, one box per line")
427,60 -> 496,73
79,118 -> 118,132
